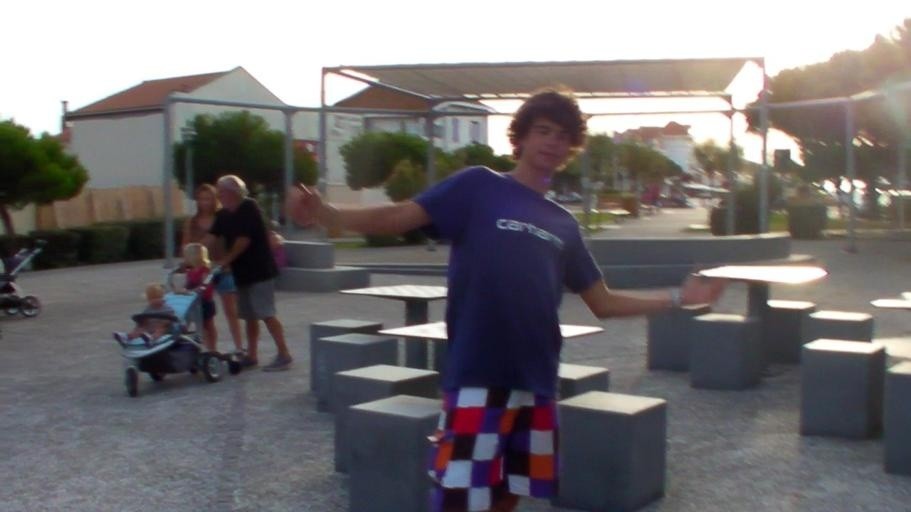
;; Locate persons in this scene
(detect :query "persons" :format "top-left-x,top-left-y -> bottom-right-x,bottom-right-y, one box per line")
174,173 -> 294,371
110,283 -> 178,349
176,240 -> 218,353
181,183 -> 243,356
284,86 -> 728,511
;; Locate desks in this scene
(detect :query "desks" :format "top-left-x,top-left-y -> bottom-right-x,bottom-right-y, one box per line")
698,264 -> 829,317
869,291 -> 911,314
380,318 -> 606,347
339,283 -> 448,371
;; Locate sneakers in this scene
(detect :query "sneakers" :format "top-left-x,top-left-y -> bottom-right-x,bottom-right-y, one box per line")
267,356 -> 294,371
238,357 -> 258,366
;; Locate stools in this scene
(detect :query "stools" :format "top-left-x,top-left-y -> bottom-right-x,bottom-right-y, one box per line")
881,363 -> 911,475
765,299 -> 817,364
806,311 -> 874,344
555,360 -> 611,393
309,318 -> 384,393
687,313 -> 770,393
800,337 -> 885,445
647,304 -> 710,371
332,361 -> 440,471
553,390 -> 668,512
343,391 -> 448,512
314,331 -> 400,413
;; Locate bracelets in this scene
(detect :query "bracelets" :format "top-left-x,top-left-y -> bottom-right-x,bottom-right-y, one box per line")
671,288 -> 681,309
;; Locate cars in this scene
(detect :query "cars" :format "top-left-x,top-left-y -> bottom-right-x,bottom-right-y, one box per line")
545,189 -> 584,204
655,196 -> 694,208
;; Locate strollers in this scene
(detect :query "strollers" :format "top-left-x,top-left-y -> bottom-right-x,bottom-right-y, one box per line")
0,236 -> 52,318
114,257 -> 230,398
